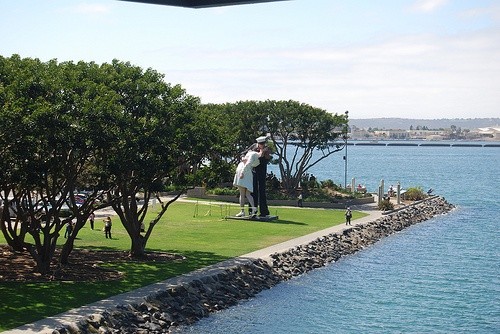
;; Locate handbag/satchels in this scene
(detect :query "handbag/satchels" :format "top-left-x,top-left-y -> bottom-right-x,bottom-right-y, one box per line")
350,214 -> 352,218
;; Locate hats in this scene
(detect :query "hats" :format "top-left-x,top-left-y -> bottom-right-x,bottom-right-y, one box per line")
347,207 -> 350,210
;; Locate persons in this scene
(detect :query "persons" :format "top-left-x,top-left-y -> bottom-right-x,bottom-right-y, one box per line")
266,169 -> 318,208
344,207 -> 352,225
63,211 -> 113,240
347,181 -> 434,196
233,136 -> 273,217
